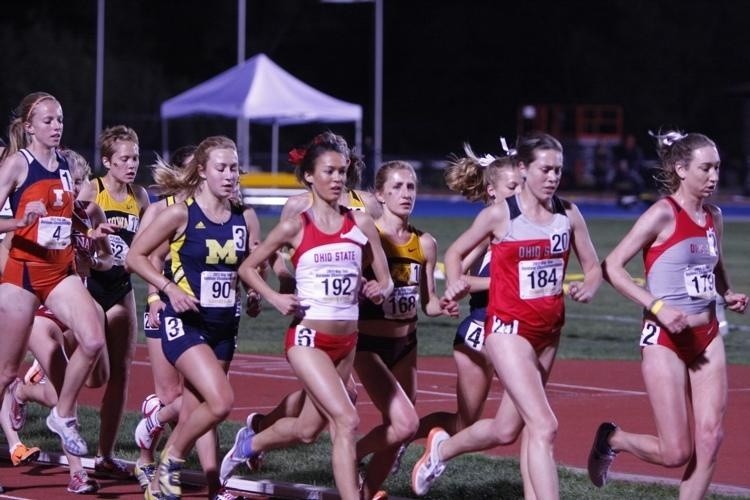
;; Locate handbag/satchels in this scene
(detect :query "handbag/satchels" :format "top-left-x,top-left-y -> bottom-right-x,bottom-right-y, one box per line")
651,298 -> 663,317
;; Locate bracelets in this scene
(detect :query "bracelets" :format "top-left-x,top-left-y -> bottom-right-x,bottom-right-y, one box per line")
147,295 -> 160,304
86,228 -> 92,237
651,298 -> 663,317
163,281 -> 173,292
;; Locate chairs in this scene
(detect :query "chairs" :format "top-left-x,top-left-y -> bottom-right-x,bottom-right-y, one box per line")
132,458 -> 160,489
23,357 -> 46,387
133,393 -> 170,450
93,453 -> 133,479
158,446 -> 187,500
368,443 -> 407,500
44,405 -> 89,457
8,443 -> 41,468
219,411 -> 267,481
410,425 -> 450,496
143,482 -> 168,500
587,421 -> 623,487
208,486 -> 247,500
66,471 -> 100,495
9,376 -> 28,432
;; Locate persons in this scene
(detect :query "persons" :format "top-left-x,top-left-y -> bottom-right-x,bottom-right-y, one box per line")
75,127 -> 153,481
218,138 -> 395,500
124,136 -> 266,500
248,132 -> 357,471
0,91 -> 122,494
351,162 -> 460,497
611,133 -> 646,211
587,129 -> 749,500
391,152 -> 526,475
413,132 -> 600,499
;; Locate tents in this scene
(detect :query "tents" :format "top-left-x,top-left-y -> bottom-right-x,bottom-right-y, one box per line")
162,54 -> 362,190
